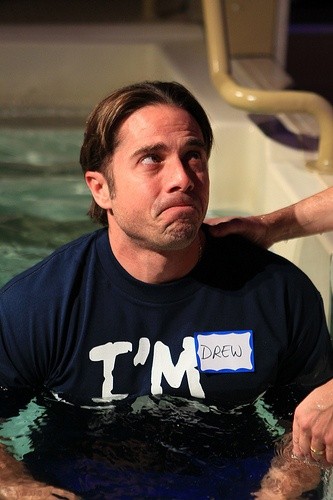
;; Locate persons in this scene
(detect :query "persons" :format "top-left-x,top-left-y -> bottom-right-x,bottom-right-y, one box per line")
0,81 -> 333,500
203,188 -> 333,468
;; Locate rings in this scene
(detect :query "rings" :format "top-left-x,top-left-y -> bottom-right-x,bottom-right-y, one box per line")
311,448 -> 326,455
